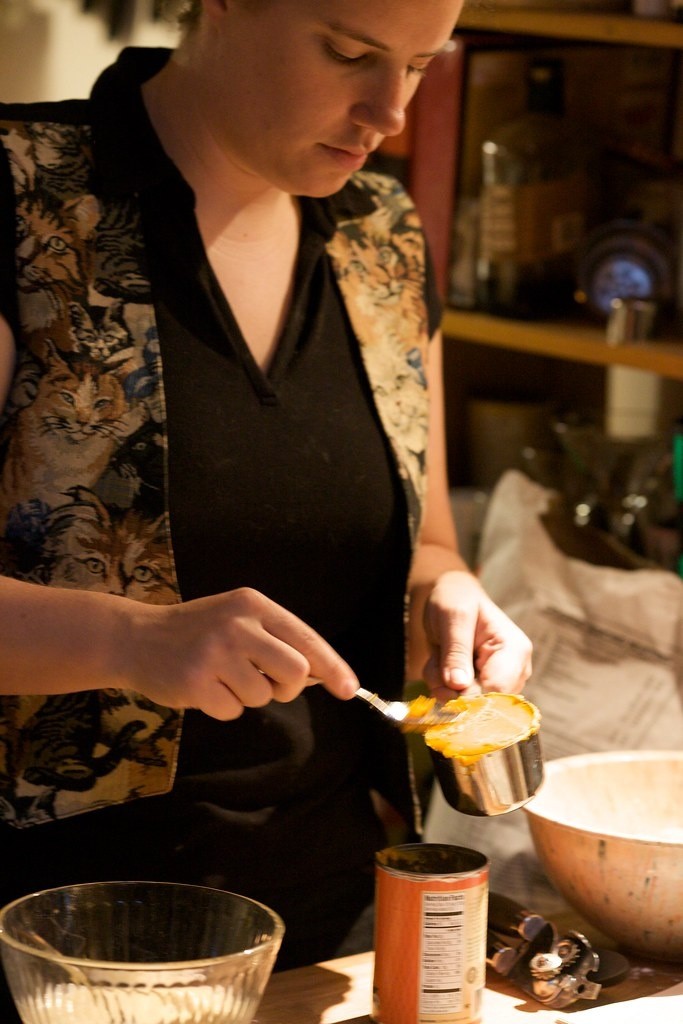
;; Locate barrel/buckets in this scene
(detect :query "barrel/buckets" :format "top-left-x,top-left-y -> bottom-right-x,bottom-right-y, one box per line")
366,842 -> 491,1024
459,389 -> 563,496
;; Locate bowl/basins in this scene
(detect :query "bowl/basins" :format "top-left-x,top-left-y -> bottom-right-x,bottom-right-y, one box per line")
0,881 -> 287,1024
521,750 -> 683,964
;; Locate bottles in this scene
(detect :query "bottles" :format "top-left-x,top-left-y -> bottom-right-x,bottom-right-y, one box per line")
479,53 -> 607,324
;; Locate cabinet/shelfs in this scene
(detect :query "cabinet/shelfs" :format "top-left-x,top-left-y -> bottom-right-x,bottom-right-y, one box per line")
398,0 -> 683,576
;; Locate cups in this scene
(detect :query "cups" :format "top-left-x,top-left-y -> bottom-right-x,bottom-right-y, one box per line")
424,678 -> 545,816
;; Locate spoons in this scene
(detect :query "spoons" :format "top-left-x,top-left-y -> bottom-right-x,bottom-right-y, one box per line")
355,687 -> 466,726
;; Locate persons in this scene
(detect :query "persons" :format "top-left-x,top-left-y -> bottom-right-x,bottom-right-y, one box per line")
0,0 -> 535,1024
